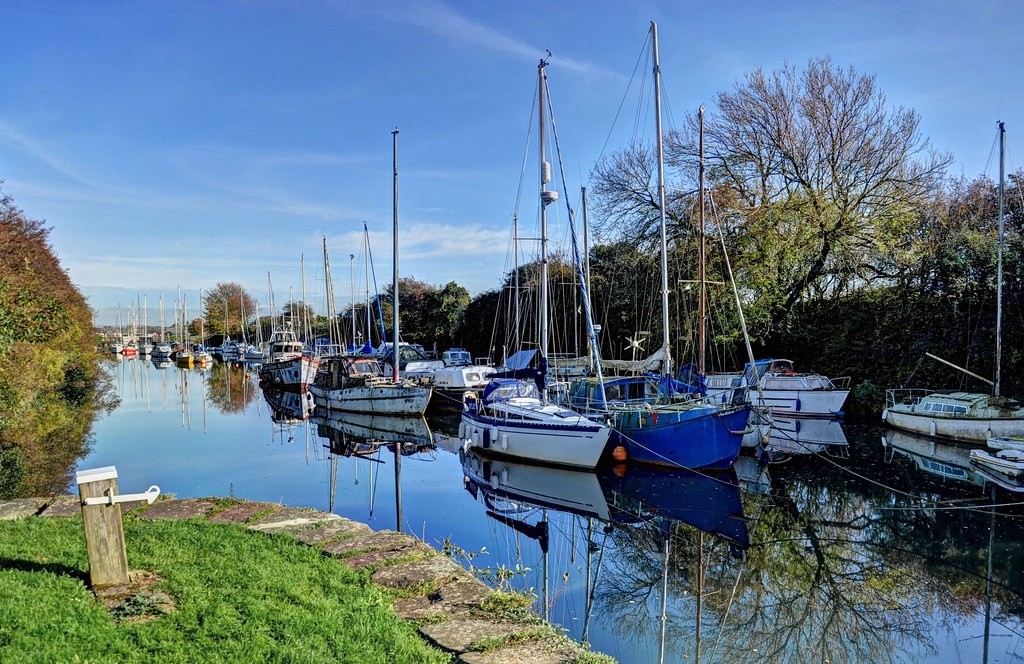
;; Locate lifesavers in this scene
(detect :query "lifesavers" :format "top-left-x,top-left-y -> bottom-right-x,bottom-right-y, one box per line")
462,391 -> 476,412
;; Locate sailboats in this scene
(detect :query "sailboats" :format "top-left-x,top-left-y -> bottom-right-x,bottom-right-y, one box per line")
106,220 -> 498,404
562,19 -> 754,470
457,49 -> 618,471
881,429 -> 997,663
879,119 -> 1023,442
459,444 -> 614,624
595,456 -> 751,663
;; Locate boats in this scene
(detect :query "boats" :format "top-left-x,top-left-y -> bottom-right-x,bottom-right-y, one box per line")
307,126 -> 434,415
259,380 -> 316,445
741,410 -> 850,459
259,321 -> 319,390
703,358 -> 853,415
309,405 -> 438,534
